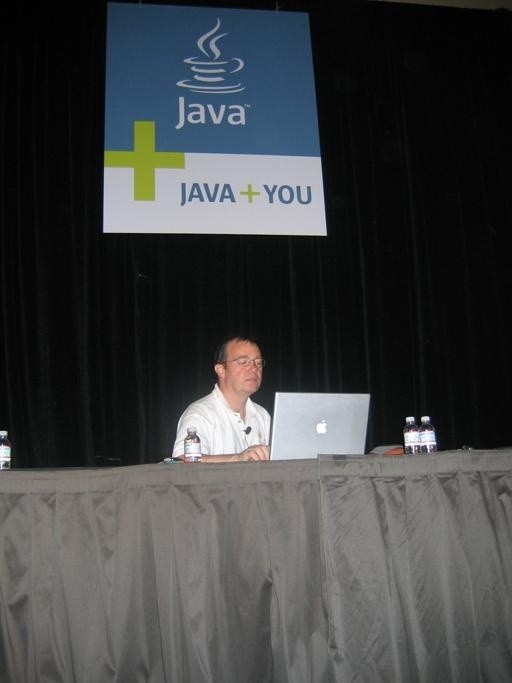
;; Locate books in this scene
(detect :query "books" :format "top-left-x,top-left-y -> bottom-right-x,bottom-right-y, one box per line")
163,456 -> 183,460
159,460 -> 184,464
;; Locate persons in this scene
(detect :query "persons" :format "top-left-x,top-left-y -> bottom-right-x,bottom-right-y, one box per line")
171,332 -> 274,463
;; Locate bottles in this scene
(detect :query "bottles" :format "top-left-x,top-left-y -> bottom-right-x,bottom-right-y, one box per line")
403,415 -> 438,454
185,427 -> 202,464
0,430 -> 12,471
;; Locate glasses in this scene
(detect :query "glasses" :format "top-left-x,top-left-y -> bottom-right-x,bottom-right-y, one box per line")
219,357 -> 266,368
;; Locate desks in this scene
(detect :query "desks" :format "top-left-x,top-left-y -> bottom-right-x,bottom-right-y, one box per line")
3,449 -> 512,680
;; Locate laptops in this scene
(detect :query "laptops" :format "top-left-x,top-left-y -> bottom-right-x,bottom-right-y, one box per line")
268,391 -> 371,461
